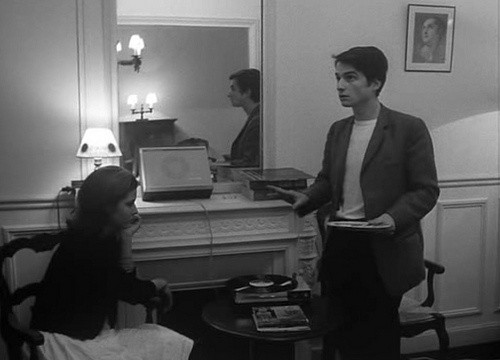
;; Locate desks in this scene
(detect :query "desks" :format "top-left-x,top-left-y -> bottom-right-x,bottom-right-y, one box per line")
72,191 -> 317,309
118,118 -> 177,165
200,290 -> 334,360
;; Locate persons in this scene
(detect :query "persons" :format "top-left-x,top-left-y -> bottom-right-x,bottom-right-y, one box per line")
227,69 -> 260,167
23,165 -> 194,360
268,46 -> 441,360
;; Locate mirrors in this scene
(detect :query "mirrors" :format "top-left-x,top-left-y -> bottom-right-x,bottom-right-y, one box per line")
102,0 -> 264,176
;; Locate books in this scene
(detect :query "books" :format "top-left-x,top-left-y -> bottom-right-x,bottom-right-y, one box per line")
253,305 -> 310,332
240,168 -> 314,201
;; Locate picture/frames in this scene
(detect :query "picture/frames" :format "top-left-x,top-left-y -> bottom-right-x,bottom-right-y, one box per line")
404,4 -> 456,73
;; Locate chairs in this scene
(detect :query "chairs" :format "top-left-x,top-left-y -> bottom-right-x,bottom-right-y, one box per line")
397,260 -> 450,360
0,229 -> 174,360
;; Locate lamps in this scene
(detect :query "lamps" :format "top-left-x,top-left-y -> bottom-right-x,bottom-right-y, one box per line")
126,93 -> 156,121
117,34 -> 145,72
76,128 -> 122,171
